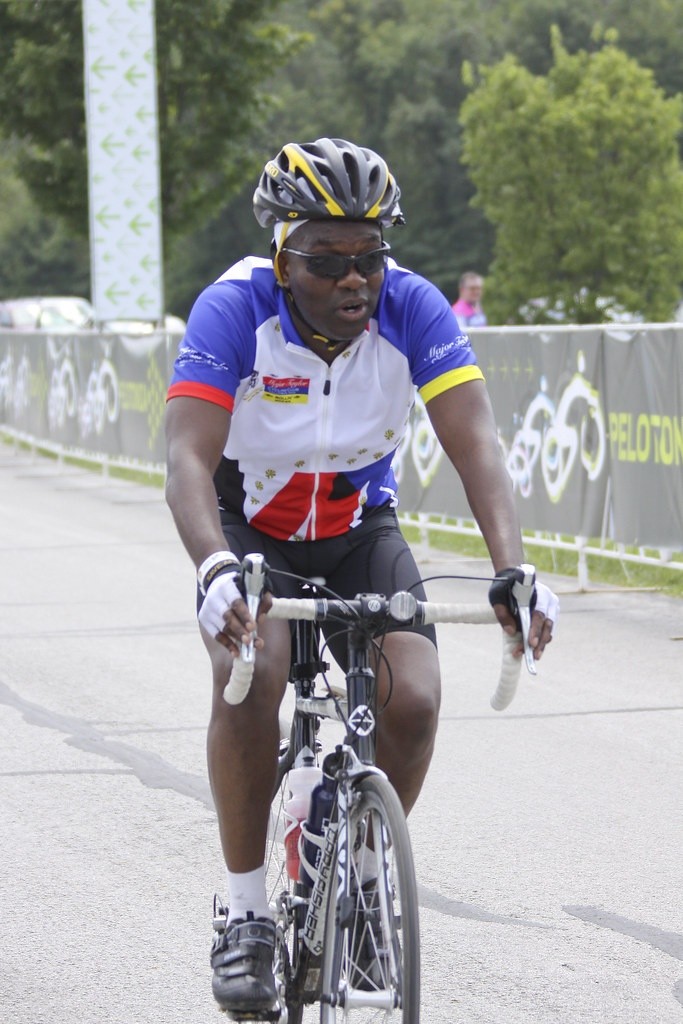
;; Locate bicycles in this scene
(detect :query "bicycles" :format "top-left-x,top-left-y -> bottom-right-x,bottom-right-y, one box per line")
219,552 -> 539,1024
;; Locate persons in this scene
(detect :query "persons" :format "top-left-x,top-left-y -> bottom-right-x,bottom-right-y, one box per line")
163,134 -> 559,1012
451,270 -> 488,327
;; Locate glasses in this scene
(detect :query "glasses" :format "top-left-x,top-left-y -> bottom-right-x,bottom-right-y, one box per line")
282,240 -> 391,279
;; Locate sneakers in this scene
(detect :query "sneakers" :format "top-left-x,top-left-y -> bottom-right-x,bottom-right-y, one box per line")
344,873 -> 403,991
210,910 -> 278,1011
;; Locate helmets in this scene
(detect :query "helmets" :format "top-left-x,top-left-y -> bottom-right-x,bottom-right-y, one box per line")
253,137 -> 406,228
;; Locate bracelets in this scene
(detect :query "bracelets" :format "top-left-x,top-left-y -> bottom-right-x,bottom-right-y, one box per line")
196,550 -> 243,597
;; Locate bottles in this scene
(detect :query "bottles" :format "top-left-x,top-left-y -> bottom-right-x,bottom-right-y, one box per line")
298,745 -> 349,890
280,756 -> 322,881
